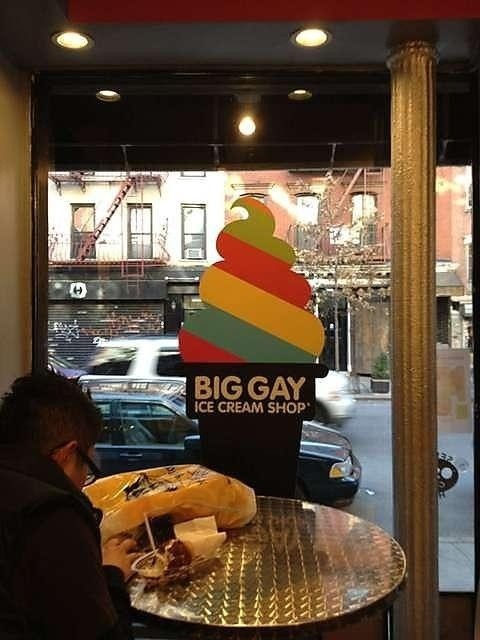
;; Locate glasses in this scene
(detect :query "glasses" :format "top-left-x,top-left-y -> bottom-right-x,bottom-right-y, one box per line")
50,439 -> 101,486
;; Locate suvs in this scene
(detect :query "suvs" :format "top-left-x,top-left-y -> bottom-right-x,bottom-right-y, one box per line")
84,394 -> 361,508
77,338 -> 357,427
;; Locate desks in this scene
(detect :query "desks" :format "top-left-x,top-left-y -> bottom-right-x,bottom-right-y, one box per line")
128,494 -> 408,639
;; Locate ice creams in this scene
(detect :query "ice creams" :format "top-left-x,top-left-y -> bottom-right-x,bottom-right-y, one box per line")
177,196 -> 329,499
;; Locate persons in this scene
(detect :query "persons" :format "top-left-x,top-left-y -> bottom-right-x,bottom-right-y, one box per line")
0,366 -> 149,639
